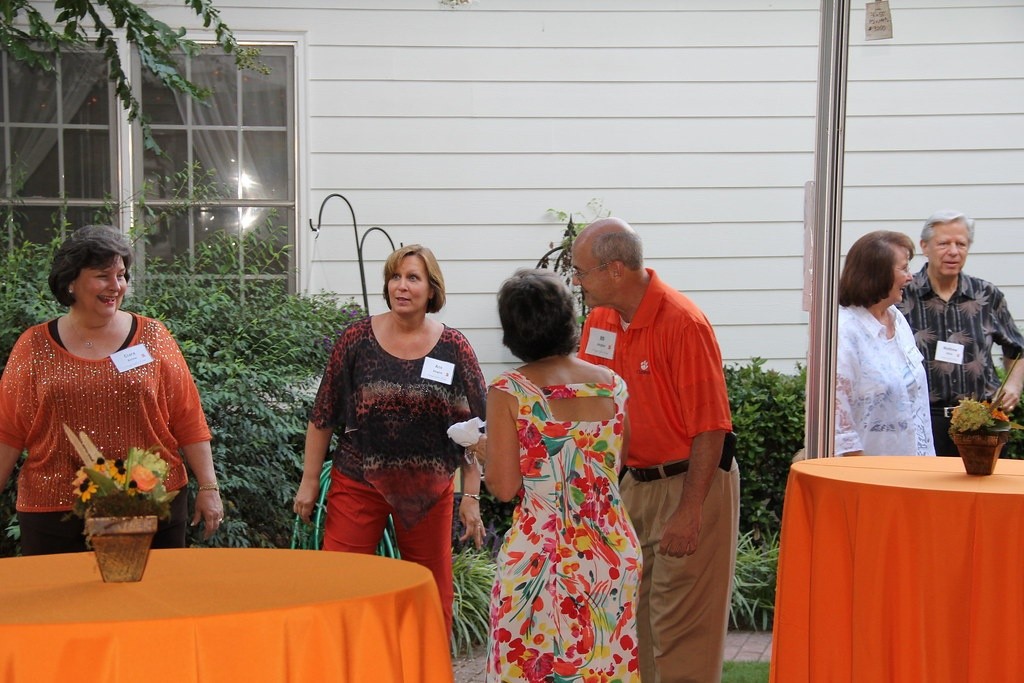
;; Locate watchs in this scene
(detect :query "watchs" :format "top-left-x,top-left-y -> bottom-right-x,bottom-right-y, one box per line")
199,482 -> 219,491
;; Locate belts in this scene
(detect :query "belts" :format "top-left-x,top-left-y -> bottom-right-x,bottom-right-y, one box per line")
930,407 -> 956,417
628,460 -> 689,482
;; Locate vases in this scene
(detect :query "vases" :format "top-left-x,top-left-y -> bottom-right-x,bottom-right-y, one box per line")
88,516 -> 159,583
953,434 -> 1006,476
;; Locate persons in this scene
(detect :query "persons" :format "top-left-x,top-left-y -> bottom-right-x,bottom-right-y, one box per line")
0,224 -> 225,556
465,266 -> 645,683
834,229 -> 937,457
293,244 -> 488,649
570,217 -> 742,683
894,208 -> 1024,457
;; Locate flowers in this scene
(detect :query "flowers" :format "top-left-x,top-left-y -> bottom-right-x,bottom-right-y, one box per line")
62,424 -> 179,551
948,352 -> 1024,445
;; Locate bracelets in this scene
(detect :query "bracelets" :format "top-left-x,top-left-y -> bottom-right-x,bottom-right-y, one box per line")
463,493 -> 480,501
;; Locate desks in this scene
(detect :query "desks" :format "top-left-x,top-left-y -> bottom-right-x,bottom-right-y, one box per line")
770,455 -> 1024,683
0,547 -> 455,683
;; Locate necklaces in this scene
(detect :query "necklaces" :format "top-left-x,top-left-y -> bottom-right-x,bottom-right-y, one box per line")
69,313 -> 113,348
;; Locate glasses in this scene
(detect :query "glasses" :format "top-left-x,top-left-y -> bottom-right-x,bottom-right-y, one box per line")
569,262 -> 612,280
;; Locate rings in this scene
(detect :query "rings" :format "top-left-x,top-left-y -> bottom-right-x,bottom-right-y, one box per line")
218,518 -> 224,524
474,523 -> 482,527
1009,406 -> 1015,410
